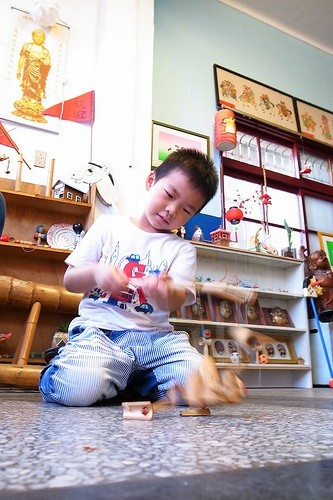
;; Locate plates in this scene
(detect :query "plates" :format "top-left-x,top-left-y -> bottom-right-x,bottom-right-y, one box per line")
46,223 -> 86,252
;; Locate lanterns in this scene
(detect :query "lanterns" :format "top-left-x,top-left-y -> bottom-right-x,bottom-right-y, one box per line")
215,109 -> 237,151
225,207 -> 243,225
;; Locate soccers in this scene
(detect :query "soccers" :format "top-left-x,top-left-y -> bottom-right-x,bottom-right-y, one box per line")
229,352 -> 240,363
258,353 -> 270,364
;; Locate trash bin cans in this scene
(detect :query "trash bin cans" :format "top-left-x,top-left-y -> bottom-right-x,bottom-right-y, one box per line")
308,315 -> 333,385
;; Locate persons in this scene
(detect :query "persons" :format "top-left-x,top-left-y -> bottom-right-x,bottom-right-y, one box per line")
37,147 -> 218,410
12,29 -> 52,123
307,248 -> 333,315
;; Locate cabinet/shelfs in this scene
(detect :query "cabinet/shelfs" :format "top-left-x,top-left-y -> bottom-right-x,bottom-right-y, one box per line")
1,177 -> 96,362
167,240 -> 313,388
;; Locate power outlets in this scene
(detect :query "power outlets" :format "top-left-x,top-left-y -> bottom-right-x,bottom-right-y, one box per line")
34,151 -> 47,168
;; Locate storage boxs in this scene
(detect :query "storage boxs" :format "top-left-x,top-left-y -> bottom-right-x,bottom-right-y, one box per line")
309,317 -> 333,385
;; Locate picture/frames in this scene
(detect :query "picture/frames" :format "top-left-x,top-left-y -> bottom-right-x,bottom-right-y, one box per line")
213,63 -> 298,132
318,231 -> 332,273
239,299 -> 264,325
258,334 -> 298,364
207,333 -> 250,363
211,295 -> 238,323
151,121 -> 210,172
186,293 -> 211,320
295,97 -> 333,145
262,307 -> 294,327
